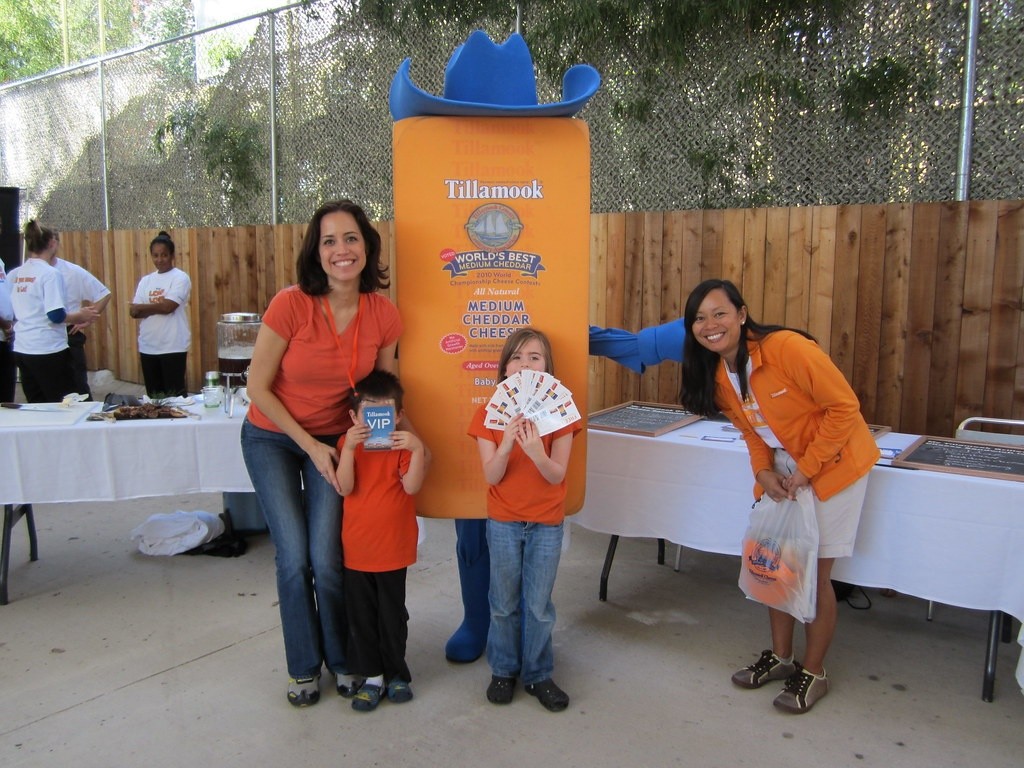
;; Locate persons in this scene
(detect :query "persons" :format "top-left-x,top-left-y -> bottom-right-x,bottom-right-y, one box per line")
335,368 -> 426,711
469,328 -> 584,711
241,197 -> 433,706
679,278 -> 881,713
0,219 -> 112,406
130,231 -> 191,398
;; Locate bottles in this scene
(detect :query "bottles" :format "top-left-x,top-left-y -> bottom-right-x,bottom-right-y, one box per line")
215,312 -> 264,391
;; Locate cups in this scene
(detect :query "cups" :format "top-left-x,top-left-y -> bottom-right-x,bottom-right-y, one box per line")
201,385 -> 224,416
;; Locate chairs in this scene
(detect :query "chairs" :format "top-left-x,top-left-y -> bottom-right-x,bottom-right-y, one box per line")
928,417 -> 1024,627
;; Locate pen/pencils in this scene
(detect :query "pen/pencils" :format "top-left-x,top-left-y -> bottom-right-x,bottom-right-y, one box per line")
705,438 -> 734,442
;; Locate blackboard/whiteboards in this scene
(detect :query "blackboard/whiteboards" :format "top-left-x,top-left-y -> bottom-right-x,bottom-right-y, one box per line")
892,435 -> 1024,482
866,424 -> 892,440
586,400 -> 705,436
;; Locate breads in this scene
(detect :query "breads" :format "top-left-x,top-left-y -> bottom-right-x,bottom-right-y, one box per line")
113,403 -> 187,420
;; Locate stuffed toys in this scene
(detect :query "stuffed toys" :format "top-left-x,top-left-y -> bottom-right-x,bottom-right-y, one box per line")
388,30 -> 685,663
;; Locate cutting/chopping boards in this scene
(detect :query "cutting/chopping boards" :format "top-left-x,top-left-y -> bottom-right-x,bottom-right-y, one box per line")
0,401 -> 100,427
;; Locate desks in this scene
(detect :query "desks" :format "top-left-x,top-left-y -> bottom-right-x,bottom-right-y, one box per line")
569,390 -> 1024,709
0,386 -> 427,611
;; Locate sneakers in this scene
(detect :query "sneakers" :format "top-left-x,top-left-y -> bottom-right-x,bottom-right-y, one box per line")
774,660 -> 827,714
731,649 -> 797,689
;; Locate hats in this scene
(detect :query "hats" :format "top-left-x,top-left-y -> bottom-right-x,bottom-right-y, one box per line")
742,536 -> 801,605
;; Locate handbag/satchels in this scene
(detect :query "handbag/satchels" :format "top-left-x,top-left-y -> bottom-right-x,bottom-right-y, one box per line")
737,483 -> 820,623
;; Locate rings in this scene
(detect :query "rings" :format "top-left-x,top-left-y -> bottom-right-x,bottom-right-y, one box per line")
320,474 -> 325,477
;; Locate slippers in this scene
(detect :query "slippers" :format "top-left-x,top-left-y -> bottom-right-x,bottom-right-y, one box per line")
352,678 -> 386,710
386,680 -> 414,703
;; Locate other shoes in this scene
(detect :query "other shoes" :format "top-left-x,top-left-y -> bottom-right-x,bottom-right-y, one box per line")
286,670 -> 322,707
487,674 -> 517,704
335,671 -> 363,698
525,678 -> 570,712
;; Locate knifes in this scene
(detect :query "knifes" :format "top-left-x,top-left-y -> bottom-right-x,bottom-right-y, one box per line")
0,403 -> 72,413
